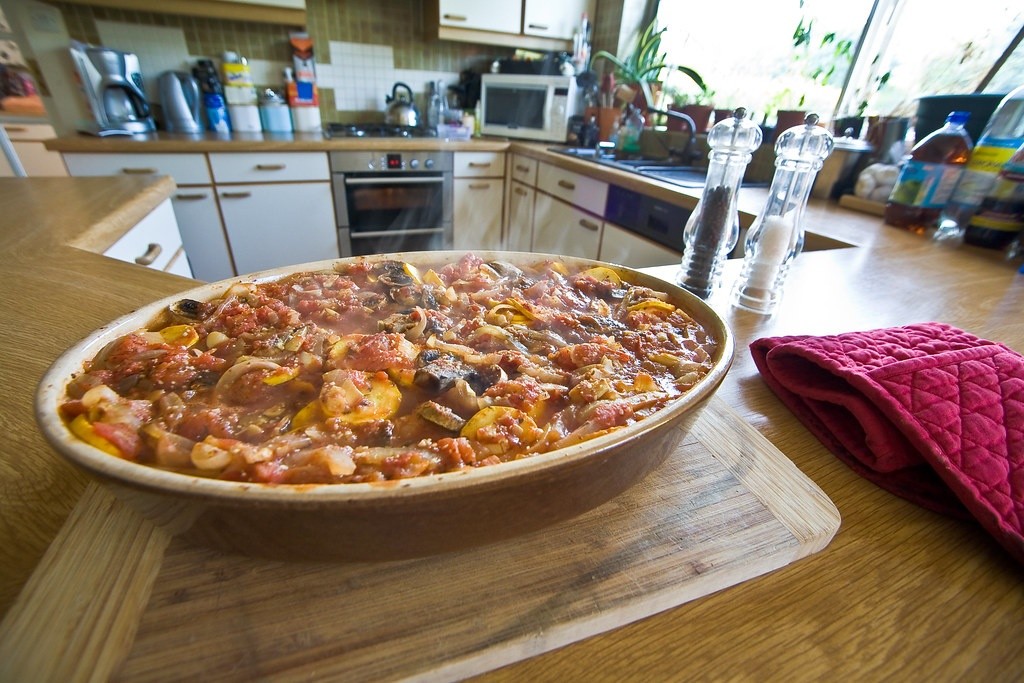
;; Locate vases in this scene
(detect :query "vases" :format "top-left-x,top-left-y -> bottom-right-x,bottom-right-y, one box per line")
715,109 -> 733,124
867,116 -> 910,146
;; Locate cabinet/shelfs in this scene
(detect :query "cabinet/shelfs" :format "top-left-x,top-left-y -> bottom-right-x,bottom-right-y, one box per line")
452,152 -> 684,269
59,151 -> 341,283
421,0 -> 598,53
0,124 -> 68,176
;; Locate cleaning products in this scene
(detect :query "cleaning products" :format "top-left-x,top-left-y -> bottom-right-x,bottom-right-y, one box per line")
607,102 -> 646,154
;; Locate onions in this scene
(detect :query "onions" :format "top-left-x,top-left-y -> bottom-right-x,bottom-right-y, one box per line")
79,281 -> 671,473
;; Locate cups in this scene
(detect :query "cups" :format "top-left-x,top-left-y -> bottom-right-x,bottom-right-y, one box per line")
810,128 -> 875,201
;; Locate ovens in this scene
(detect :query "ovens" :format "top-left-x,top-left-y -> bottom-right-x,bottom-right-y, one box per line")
327,151 -> 453,258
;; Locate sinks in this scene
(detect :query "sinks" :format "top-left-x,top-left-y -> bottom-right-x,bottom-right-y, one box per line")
638,166 -> 758,185
614,159 -> 691,168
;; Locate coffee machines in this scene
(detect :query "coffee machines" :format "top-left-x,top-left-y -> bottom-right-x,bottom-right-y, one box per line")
70,46 -> 156,134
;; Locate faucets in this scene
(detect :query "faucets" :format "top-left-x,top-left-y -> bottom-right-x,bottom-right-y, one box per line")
646,105 -> 698,165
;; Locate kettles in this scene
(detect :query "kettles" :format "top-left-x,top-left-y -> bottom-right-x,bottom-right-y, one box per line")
157,70 -> 207,133
384,82 -> 419,127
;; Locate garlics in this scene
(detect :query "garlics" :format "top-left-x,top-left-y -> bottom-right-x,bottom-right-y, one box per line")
855,164 -> 899,200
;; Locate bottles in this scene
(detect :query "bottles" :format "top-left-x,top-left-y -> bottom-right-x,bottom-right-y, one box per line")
934,82 -> 1024,252
227,103 -> 322,133
729,113 -> 835,315
605,116 -> 621,154
675,108 -> 764,300
570,115 -> 584,146
584,80 -> 599,125
205,95 -> 230,133
884,111 -> 974,235
584,114 -> 600,149
194,59 -> 223,95
621,104 -> 645,152
426,81 -> 441,130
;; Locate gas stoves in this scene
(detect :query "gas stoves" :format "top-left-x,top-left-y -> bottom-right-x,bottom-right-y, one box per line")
322,123 -> 437,140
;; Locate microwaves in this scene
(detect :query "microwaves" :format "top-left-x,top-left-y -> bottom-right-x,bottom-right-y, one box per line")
480,74 -> 577,142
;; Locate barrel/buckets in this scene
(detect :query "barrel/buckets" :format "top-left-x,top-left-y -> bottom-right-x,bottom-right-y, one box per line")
913,94 -> 1011,150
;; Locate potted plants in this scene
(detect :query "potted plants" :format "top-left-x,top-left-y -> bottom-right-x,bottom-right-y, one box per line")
589,18 -> 714,127
764,14 -> 892,141
664,84 -> 711,134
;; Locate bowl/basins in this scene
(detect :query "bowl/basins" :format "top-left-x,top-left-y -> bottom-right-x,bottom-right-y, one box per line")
33,251 -> 734,562
